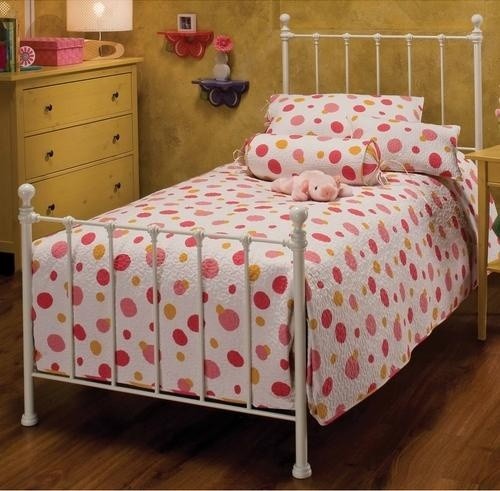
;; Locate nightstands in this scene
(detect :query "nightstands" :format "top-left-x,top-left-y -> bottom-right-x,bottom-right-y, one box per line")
465,144 -> 500,340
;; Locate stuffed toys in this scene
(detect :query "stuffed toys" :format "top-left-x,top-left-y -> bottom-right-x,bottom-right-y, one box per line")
268,166 -> 353,204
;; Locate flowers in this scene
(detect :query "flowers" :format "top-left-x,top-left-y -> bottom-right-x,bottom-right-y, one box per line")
214,33 -> 233,54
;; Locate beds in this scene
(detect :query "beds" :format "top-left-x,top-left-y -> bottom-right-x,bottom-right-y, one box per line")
16,14 -> 483,480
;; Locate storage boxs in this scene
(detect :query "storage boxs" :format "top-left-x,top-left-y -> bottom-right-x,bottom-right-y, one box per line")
20,37 -> 85,67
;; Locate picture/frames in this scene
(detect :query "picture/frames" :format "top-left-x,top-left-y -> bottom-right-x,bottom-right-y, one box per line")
0,17 -> 20,73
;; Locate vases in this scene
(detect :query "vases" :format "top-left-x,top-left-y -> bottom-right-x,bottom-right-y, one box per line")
214,50 -> 231,82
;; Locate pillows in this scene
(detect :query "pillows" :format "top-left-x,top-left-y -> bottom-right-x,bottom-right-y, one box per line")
230,133 -> 389,187
354,116 -> 464,182
267,93 -> 425,123
264,113 -> 354,140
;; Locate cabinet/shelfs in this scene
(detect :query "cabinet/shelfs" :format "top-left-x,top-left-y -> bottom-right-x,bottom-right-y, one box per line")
156,29 -> 250,108
0,56 -> 144,276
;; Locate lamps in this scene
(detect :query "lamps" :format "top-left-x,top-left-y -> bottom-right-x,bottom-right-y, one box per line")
66,0 -> 134,56
